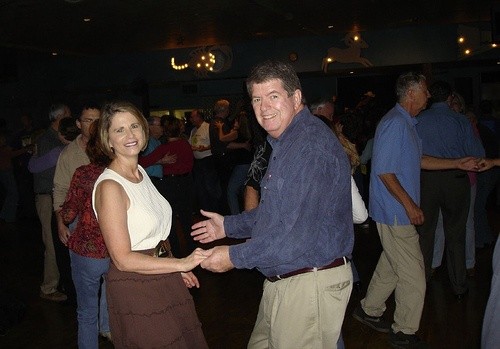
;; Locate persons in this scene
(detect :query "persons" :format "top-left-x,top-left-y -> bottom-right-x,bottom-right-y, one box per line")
476,157 -> 500,349
52,103 -> 101,246
242,81 -> 500,349
0,105 -> 83,302
137,98 -> 274,264
190,58 -> 355,349
92,101 -> 211,349
352,73 -> 481,349
61,119 -> 115,349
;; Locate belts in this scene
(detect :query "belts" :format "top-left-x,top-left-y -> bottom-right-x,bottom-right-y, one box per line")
267,256 -> 350,282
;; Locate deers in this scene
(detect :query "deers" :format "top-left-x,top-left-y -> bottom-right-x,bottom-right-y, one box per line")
320,29 -> 374,73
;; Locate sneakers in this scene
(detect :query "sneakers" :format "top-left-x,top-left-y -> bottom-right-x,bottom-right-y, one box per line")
353,308 -> 389,333
391,331 -> 412,349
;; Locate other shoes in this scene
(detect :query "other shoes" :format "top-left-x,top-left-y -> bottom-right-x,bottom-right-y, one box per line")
41,287 -> 67,300
